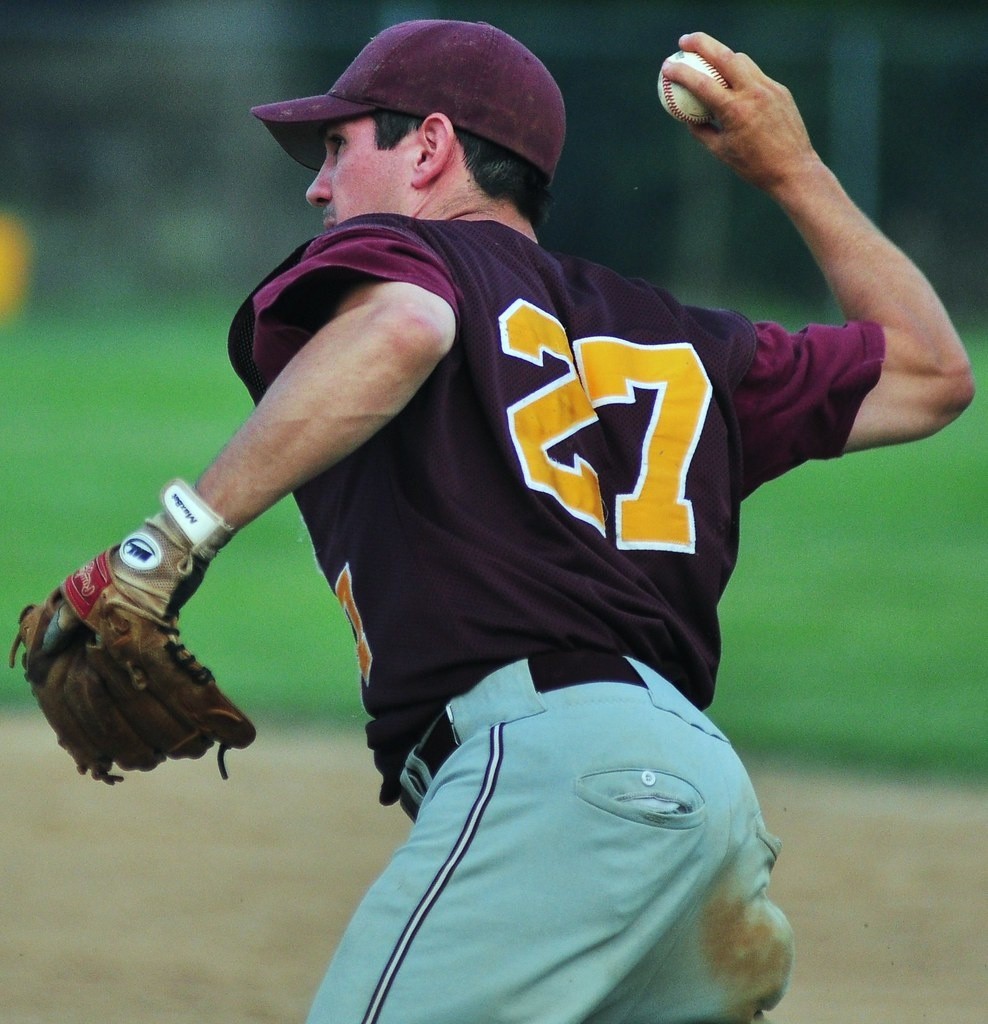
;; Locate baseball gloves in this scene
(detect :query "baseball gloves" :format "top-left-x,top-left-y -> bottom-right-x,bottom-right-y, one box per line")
6,474 -> 258,790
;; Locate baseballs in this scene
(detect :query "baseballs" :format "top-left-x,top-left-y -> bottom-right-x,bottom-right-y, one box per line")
654,49 -> 733,127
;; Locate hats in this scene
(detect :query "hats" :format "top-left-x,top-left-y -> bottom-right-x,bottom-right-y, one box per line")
249,19 -> 566,187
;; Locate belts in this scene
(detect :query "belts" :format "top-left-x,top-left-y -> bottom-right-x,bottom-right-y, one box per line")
398,652 -> 646,822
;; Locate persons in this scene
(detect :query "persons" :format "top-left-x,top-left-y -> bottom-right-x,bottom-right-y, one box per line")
8,19 -> 978,1022
